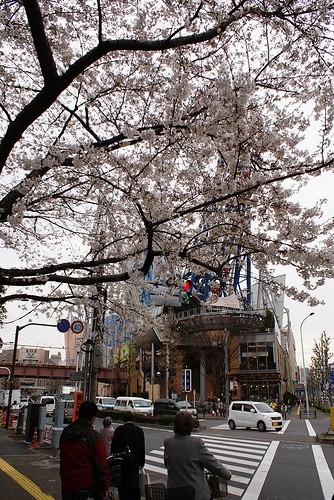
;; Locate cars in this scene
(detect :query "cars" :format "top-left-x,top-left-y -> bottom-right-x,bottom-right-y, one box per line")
144,399 -> 155,411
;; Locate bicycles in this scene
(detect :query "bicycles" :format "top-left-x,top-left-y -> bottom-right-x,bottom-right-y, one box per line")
145,468 -> 232,500
94,449 -> 132,500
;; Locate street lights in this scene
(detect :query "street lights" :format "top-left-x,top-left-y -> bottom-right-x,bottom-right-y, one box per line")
300,312 -> 315,418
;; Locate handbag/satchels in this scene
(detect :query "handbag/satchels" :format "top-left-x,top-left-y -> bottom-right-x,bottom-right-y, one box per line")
139,466 -> 152,499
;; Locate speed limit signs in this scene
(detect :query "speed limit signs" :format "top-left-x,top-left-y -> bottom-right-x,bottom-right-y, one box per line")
71,320 -> 84,334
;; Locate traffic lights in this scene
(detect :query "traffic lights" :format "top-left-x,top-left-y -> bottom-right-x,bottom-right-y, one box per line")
232,381 -> 238,391
178,279 -> 192,312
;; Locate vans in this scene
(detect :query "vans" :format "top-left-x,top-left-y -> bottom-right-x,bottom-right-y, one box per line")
20,396 -> 74,424
113,396 -> 152,417
95,396 -> 116,410
152,398 -> 199,418
227,401 -> 283,432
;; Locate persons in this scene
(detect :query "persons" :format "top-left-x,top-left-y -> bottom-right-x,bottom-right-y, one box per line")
110,411 -> 145,500
101,417 -> 116,455
201,400 -> 225,418
286,399 -> 292,412
270,400 -> 277,410
163,412 -> 231,500
297,399 -> 300,407
12,401 -> 21,407
281,403 -> 287,420
59,401 -> 110,500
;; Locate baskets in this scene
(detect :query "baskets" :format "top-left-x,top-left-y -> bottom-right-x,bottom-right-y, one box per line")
206,473 -> 228,498
